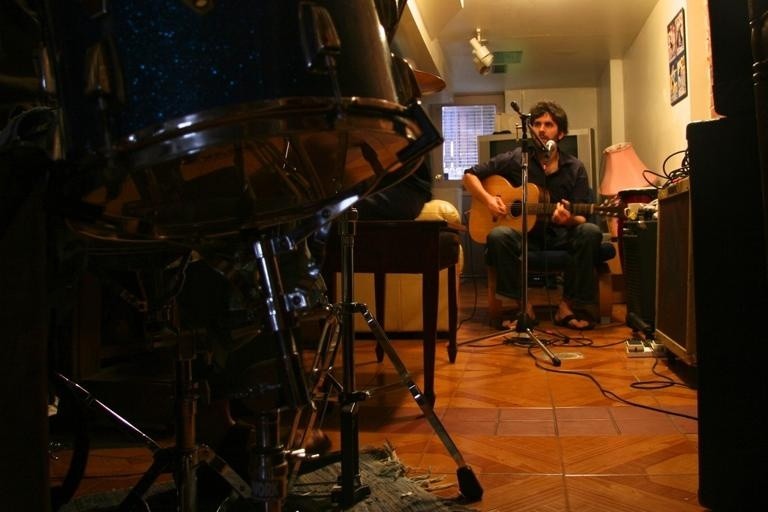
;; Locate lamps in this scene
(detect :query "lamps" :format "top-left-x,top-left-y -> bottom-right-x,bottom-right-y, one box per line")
470,29 -> 494,76
596,142 -> 658,242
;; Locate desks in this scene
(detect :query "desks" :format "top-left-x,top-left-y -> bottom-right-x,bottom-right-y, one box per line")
323,219 -> 464,413
620,217 -> 657,336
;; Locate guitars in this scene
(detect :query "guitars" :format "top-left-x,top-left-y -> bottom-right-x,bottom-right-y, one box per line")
468,174 -> 626,244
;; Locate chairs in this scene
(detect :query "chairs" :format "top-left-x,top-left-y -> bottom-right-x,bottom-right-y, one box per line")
487,188 -> 614,328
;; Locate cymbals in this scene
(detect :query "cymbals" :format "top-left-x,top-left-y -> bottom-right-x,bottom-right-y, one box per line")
412,70 -> 446,95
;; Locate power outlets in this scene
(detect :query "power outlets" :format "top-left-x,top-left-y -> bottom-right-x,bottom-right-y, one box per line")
623,336 -> 650,358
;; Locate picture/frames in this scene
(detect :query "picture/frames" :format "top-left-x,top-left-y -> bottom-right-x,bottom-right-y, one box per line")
665,9 -> 688,106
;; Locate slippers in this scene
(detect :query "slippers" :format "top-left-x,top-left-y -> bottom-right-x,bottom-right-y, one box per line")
553,312 -> 593,331
497,308 -> 539,331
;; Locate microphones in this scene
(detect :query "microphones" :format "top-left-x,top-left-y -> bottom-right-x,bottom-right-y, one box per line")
543,140 -> 557,165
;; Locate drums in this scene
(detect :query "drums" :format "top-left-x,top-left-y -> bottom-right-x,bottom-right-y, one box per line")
62,0 -> 428,244
149,239 -> 331,348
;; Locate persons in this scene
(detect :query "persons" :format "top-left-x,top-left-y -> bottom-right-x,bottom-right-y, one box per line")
668,19 -> 686,98
460,100 -> 604,331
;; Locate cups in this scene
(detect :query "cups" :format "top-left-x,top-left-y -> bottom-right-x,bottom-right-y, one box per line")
624,202 -> 644,220
635,205 -> 654,222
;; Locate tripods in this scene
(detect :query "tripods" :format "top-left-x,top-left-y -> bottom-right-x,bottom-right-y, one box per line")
283,206 -> 484,505
116,363 -> 253,512
447,101 -> 570,367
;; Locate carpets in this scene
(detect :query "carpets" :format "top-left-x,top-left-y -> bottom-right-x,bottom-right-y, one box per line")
70,446 -> 474,511
